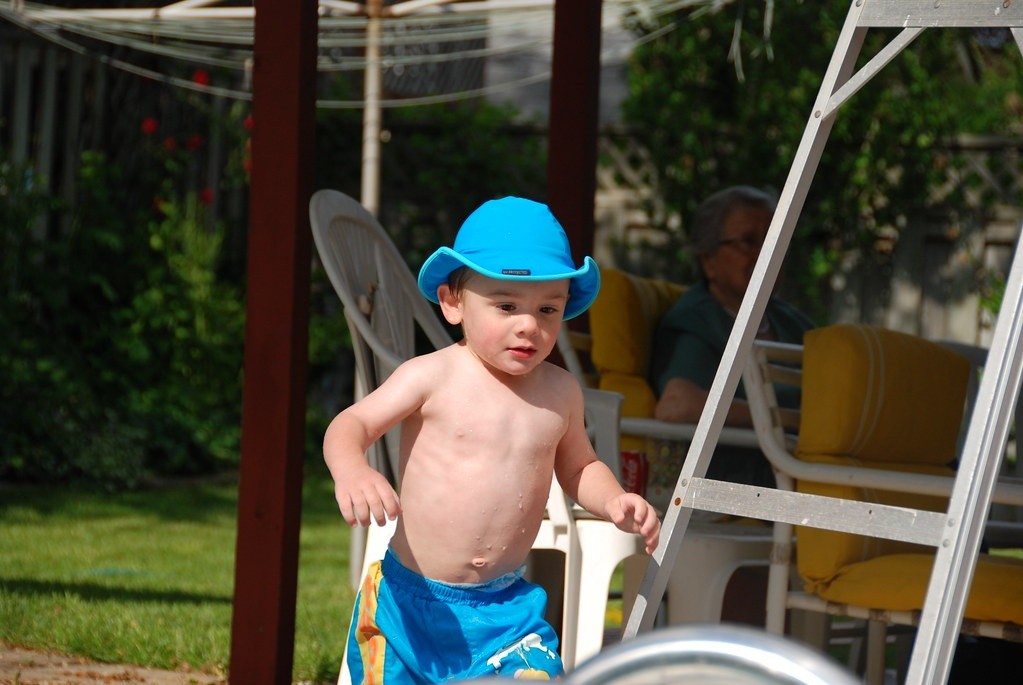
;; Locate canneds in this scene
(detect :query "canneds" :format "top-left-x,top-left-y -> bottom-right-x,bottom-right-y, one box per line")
620,450 -> 648,498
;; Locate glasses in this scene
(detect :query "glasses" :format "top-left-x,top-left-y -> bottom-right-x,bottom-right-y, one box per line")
716,232 -> 762,256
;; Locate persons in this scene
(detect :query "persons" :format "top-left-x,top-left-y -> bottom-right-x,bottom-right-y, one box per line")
323,195 -> 662,684
650,185 -> 817,489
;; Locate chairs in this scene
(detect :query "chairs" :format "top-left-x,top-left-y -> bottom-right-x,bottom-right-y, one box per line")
310,189 -> 662,685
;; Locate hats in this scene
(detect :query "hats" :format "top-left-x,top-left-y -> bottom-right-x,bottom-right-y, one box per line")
418,196 -> 601,321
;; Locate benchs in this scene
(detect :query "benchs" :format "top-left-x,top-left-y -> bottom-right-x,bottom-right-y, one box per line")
554,320 -> 829,649
740,326 -> 1023,685
